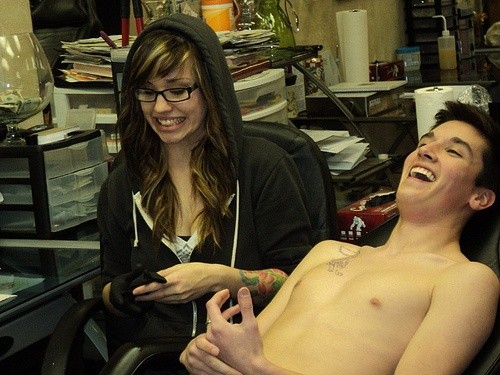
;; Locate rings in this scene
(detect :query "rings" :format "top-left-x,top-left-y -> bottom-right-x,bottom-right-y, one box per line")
205,321 -> 211,325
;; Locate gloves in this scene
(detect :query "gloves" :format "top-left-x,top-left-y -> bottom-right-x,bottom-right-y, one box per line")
109,264 -> 155,317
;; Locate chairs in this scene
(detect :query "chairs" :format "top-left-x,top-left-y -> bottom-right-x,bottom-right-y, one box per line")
42,120 -> 337,375
104,198 -> 499,375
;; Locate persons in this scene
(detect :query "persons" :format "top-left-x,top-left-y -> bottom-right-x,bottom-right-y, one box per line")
97,13 -> 315,375
179,100 -> 500,375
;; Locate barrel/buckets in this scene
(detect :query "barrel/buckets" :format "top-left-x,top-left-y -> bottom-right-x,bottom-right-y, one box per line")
201,0 -> 242,33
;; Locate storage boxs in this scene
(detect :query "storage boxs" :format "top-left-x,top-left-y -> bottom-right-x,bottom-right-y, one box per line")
0,128 -> 112,280
338,188 -> 400,239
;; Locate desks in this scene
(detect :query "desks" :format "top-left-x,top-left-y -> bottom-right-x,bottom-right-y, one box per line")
289,54 -> 500,156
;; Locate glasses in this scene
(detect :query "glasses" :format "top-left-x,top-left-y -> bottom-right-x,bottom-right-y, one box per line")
132,82 -> 201,103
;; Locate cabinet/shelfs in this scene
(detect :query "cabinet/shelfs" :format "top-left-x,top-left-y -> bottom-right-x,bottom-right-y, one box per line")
53,69 -> 289,153
404,0 -> 461,66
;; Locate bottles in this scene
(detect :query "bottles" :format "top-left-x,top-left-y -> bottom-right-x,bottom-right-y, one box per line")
432,15 -> 457,69
396,46 -> 421,72
